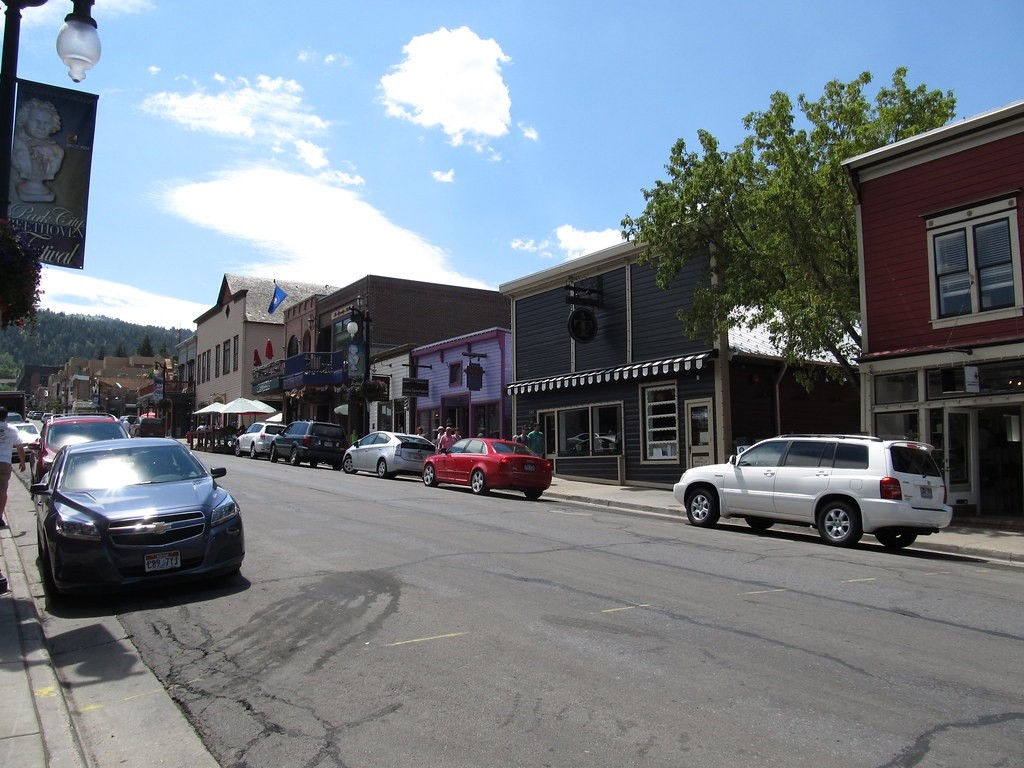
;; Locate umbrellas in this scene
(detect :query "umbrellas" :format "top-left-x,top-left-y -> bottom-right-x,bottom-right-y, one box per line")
193,398 -> 276,427
265,339 -> 274,363
254,348 -> 262,370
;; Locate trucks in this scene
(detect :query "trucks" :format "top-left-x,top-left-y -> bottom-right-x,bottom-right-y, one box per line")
0,391 -> 26,423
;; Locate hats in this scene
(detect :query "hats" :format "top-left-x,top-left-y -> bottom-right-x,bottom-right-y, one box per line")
438,426 -> 443,430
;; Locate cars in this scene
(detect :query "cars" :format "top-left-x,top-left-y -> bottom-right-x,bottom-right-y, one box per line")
119,416 -> 136,432
566,432 -> 616,452
342,431 -> 436,479
3,412 -> 42,462
28,410 -> 63,423
422,437 -> 552,501
29,435 -> 246,604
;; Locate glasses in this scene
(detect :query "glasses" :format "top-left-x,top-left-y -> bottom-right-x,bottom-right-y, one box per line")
525,429 -> 528,430
455,430 -> 459,431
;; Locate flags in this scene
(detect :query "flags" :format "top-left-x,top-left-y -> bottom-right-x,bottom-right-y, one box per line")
267,284 -> 288,314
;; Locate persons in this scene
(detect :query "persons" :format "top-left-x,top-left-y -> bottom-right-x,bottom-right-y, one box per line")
0,407 -> 26,526
12,98 -> 64,181
350,430 -> 358,446
215,422 -> 222,446
123,418 -> 131,433
477,428 -> 486,438
433,426 -> 461,454
196,423 -> 211,444
240,425 -> 247,431
186,428 -> 192,444
415,427 -> 426,443
512,423 -> 545,459
225,425 -> 237,446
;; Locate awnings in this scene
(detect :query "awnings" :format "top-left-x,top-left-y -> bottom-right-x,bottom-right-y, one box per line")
506,354 -> 710,396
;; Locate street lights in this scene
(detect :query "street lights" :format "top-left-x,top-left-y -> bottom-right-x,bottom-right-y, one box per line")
92,382 -> 100,405
348,305 -> 368,437
154,362 -> 166,416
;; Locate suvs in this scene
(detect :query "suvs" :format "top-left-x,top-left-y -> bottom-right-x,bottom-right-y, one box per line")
129,417 -> 165,438
673,433 -> 953,548
269,420 -> 349,471
28,410 -> 130,484
235,422 -> 287,460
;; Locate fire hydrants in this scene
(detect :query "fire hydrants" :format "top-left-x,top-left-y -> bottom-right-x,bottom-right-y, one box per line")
186,432 -> 191,443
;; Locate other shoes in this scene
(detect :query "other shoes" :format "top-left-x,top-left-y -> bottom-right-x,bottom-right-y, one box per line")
0,519 -> 5,527
